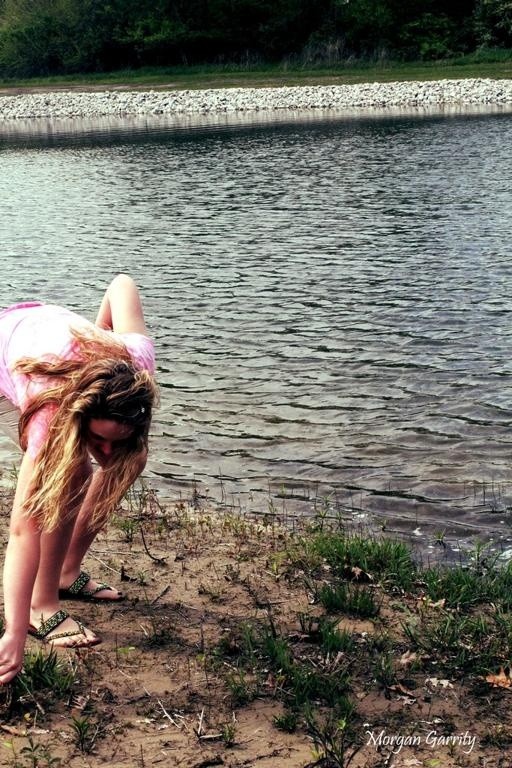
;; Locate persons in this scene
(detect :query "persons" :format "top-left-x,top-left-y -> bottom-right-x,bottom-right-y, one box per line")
0,272 -> 161,685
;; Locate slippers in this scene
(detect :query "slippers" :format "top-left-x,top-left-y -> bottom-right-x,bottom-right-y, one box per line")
28,608 -> 101,648
59,571 -> 122,603
